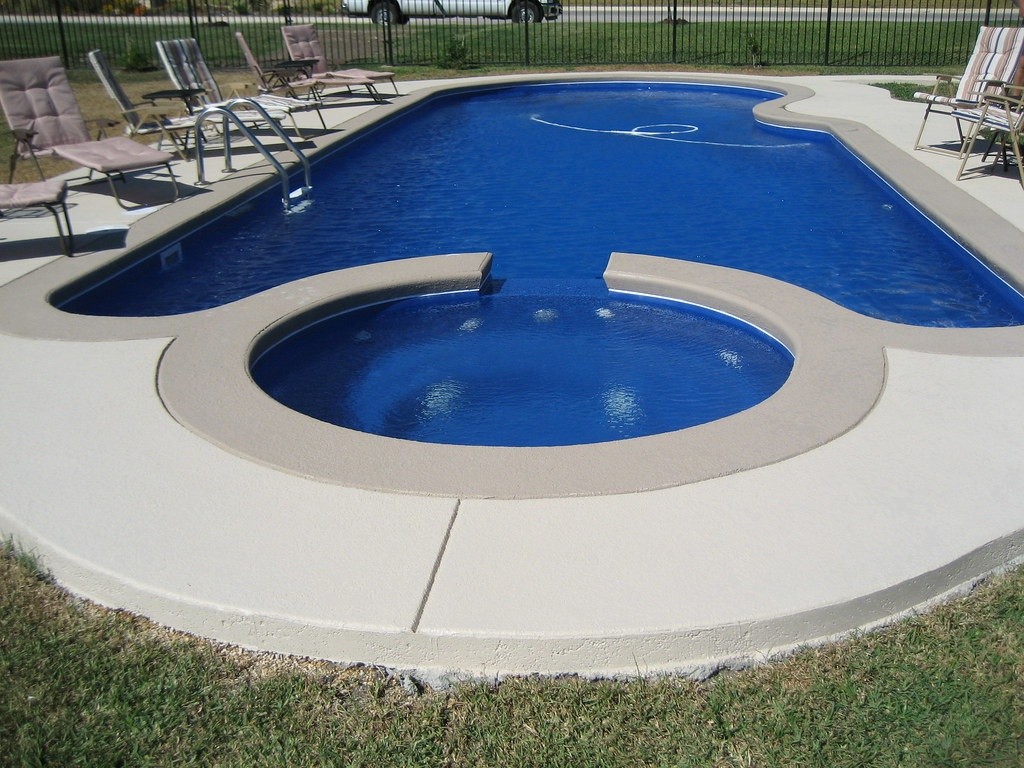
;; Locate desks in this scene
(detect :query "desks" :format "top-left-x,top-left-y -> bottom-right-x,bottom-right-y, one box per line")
276,60 -> 321,101
141,87 -> 207,155
981,94 -> 1024,172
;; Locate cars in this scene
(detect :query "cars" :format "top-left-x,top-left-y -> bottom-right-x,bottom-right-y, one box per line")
341,1 -> 566,28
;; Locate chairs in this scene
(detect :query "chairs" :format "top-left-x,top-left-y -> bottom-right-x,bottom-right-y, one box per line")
179,37 -> 326,139
912,25 -> 1024,161
156,37 -> 287,151
281,24 -> 399,104
235,32 -> 384,108
0,180 -> 73,257
951,83 -> 1024,190
88,49 -> 207,163
0,55 -> 179,211
